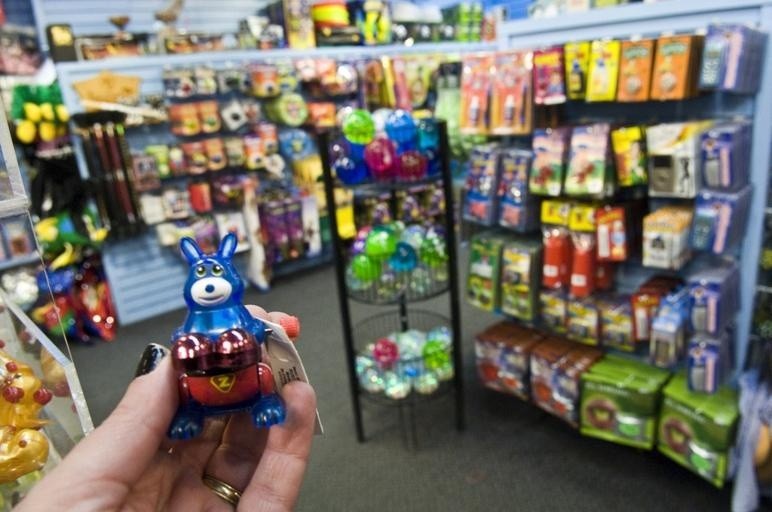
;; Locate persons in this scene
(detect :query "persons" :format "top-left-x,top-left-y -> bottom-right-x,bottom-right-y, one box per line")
11,305 -> 315,511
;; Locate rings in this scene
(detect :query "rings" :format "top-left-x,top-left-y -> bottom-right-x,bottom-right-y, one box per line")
202,469 -> 243,505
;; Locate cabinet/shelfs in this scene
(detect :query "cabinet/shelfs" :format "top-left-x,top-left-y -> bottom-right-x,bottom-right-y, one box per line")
317,120 -> 476,449
